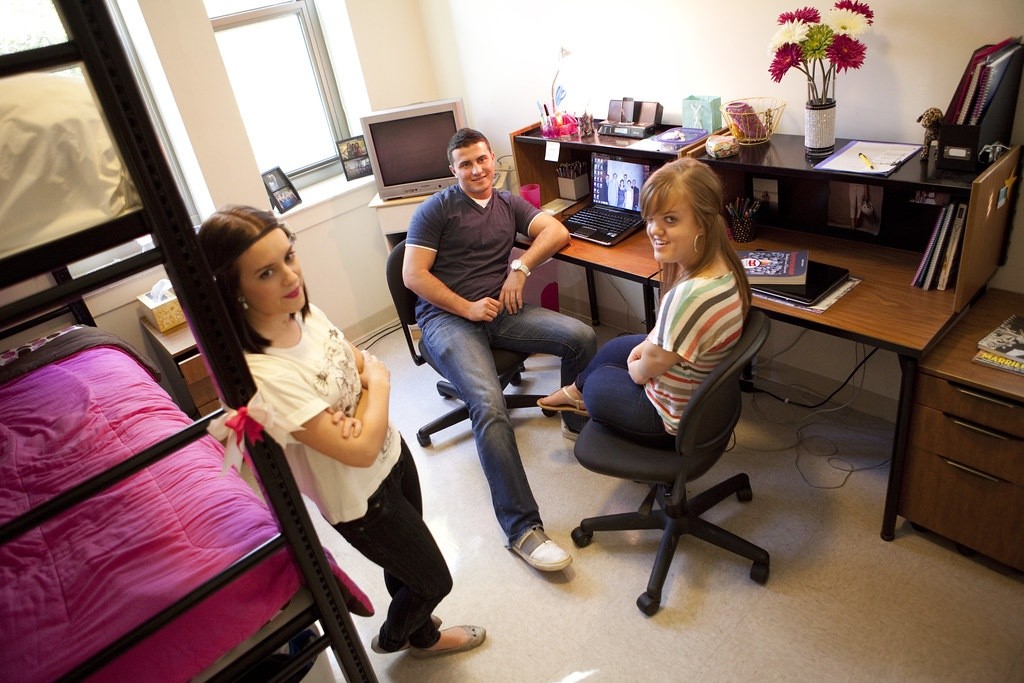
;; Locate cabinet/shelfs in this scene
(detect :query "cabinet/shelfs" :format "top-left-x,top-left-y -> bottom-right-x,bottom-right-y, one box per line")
139,315 -> 221,420
367,164 -> 514,341
897,288 -> 1024,579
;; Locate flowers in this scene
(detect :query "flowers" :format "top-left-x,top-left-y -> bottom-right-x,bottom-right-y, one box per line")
768,0 -> 876,106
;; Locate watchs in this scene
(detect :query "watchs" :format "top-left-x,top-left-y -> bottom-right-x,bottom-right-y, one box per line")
510,259 -> 531,277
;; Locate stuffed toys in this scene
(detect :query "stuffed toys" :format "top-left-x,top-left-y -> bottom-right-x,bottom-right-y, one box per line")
917,108 -> 943,160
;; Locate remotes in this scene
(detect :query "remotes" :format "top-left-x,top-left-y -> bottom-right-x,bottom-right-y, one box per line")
492,173 -> 500,186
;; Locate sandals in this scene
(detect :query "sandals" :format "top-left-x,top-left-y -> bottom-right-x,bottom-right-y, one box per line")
537,385 -> 591,417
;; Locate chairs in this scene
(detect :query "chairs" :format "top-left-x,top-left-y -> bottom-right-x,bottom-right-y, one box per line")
386,237 -> 558,449
569,311 -> 770,614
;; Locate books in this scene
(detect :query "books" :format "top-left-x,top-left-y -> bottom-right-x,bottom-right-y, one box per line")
910,203 -> 968,291
735,250 -> 808,284
971,314 -> 1024,376
956,42 -> 1023,126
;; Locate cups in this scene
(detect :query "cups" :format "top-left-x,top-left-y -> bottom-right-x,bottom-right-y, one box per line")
519,184 -> 541,210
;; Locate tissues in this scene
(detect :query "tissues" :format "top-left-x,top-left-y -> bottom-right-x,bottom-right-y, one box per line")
136,279 -> 188,333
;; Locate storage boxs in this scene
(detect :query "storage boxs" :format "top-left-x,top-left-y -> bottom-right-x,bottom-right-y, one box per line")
558,173 -> 590,199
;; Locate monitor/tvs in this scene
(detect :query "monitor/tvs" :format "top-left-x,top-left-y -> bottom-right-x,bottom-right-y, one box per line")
360,97 -> 467,201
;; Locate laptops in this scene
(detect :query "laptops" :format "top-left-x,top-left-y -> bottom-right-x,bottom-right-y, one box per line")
562,152 -> 653,246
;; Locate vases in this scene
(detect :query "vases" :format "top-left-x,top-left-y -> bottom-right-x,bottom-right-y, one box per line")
806,99 -> 836,159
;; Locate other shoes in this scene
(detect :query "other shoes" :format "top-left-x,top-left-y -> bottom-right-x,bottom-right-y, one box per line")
511,524 -> 573,572
411,624 -> 487,657
371,616 -> 442,654
559,419 -> 580,441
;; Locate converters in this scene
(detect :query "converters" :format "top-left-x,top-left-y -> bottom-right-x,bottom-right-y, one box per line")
739,379 -> 754,392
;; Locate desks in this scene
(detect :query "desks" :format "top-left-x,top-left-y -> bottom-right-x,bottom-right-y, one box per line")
510,118 -> 1024,542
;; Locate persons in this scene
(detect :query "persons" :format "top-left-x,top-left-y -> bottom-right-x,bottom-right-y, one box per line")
537,154 -> 752,450
267,174 -> 279,191
347,142 -> 362,159
601,173 -> 640,210
199,204 -> 486,658
278,193 -> 296,207
402,128 -> 598,570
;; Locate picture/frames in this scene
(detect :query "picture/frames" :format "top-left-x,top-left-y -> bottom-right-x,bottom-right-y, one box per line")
336,135 -> 373,181
261,166 -> 301,215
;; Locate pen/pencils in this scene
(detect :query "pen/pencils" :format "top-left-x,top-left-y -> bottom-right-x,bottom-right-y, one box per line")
725,198 -> 761,218
858,153 -> 873,170
556,160 -> 588,179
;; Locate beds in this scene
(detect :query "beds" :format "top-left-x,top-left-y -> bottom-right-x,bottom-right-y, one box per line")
0,0 -> 377,683
